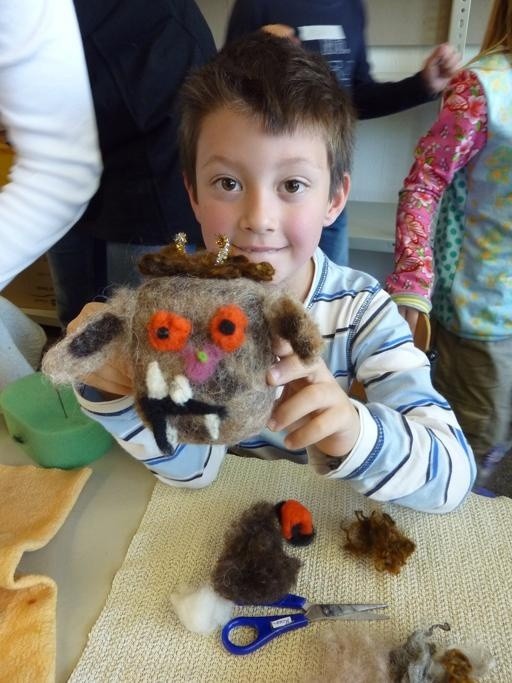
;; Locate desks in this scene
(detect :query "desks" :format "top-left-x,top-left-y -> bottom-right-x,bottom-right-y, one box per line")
346,200 -> 399,252
0,413 -> 512,682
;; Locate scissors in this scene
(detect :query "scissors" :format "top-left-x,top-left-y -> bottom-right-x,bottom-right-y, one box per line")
221,594 -> 390,654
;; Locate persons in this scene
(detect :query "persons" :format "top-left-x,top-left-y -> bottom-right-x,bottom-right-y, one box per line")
64,32 -> 479,516
0,0 -> 103,297
385,0 -> 512,488
225,0 -> 464,266
43,0 -> 218,332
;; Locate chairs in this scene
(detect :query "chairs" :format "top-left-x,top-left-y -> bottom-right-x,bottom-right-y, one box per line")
349,308 -> 439,403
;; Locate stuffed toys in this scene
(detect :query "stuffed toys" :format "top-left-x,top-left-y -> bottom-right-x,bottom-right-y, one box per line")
40,232 -> 324,456
